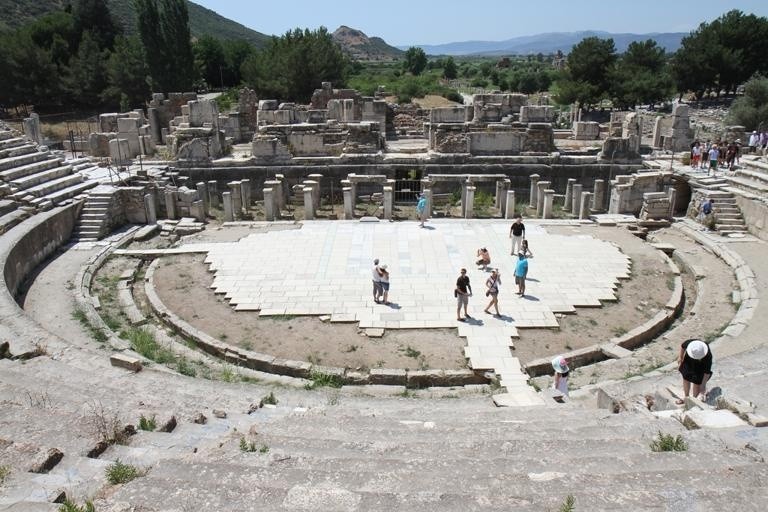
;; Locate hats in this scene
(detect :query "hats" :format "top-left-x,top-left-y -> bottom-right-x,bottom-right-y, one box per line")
552,356 -> 569,374
686,341 -> 708,360
492,268 -> 498,272
519,250 -> 524,256
374,259 -> 387,269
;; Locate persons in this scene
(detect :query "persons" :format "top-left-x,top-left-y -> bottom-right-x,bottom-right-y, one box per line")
372,258 -> 382,304
378,264 -> 390,305
518,239 -> 533,278
513,249 -> 528,298
702,198 -> 714,228
550,355 -> 571,400
456,268 -> 472,321
475,246 -> 490,272
510,215 -> 525,255
484,267 -> 501,318
689,129 -> 768,179
679,338 -> 713,398
414,192 -> 427,227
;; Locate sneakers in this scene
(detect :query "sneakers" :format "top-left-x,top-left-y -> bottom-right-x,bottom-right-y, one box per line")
497,313 -> 501,317
375,300 -> 390,304
465,314 -> 470,318
457,319 -> 465,321
485,310 -> 490,313
